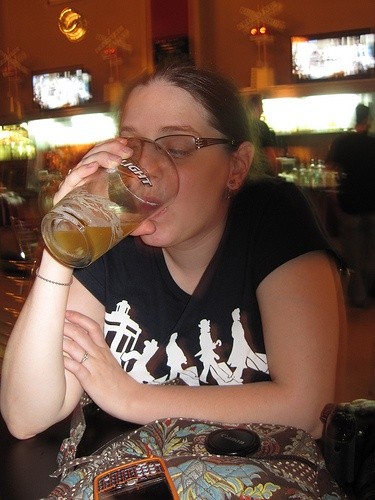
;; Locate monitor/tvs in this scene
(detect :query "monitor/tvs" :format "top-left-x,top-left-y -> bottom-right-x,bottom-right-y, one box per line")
290,30 -> 375,82
32,66 -> 94,111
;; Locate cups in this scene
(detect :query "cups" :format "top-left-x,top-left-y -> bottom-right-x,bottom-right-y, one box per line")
40,135 -> 180,270
290,167 -> 339,189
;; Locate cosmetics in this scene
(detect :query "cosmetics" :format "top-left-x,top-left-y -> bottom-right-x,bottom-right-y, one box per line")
205,428 -> 260,456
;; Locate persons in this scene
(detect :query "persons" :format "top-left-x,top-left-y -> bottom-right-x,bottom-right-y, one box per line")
246,93 -> 277,175
0,68 -> 342,469
325,103 -> 375,309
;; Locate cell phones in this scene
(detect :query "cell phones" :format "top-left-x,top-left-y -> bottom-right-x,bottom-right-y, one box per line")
93,458 -> 182,500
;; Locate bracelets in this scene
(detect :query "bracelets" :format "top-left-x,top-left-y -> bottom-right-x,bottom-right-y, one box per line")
35,266 -> 73,286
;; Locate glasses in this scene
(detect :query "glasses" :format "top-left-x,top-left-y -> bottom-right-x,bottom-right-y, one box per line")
120,133 -> 235,165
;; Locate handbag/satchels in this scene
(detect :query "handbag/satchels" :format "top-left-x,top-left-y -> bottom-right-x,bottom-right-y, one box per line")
40,417 -> 347,500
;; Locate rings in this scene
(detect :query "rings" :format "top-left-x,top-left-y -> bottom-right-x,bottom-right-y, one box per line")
81,352 -> 88,362
